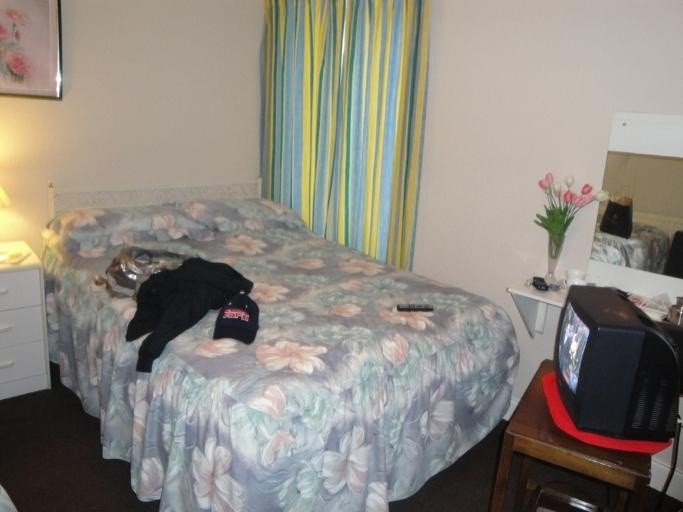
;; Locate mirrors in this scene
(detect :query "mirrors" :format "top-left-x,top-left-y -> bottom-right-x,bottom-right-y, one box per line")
583,111 -> 682,314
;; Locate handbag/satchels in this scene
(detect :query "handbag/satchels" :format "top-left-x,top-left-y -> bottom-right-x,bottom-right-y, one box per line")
599,194 -> 636,238
95,245 -> 194,298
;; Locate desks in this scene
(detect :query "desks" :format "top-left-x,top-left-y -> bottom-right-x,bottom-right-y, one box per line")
485,355 -> 655,511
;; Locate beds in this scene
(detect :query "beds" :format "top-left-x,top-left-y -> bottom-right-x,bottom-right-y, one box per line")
591,212 -> 671,277
42,179 -> 522,511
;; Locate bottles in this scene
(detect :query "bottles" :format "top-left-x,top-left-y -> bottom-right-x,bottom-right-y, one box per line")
666,296 -> 683,325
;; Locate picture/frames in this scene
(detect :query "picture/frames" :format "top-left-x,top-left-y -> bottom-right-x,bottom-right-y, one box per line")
0,0 -> 62,101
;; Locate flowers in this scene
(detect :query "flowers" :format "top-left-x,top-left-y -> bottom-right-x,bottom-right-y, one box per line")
0,5 -> 35,89
533,169 -> 614,263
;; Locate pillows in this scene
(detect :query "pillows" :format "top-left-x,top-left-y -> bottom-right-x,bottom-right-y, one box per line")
42,203 -> 213,264
161,193 -> 312,234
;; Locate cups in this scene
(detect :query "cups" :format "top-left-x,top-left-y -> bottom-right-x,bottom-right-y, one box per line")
563,269 -> 588,289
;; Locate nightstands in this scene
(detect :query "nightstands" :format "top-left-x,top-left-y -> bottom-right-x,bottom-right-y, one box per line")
0,240 -> 54,409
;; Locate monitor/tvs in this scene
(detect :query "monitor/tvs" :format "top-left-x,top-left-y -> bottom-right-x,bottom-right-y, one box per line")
554,285 -> 683,442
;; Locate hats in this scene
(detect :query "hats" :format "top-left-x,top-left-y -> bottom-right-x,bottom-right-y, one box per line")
212,289 -> 259,345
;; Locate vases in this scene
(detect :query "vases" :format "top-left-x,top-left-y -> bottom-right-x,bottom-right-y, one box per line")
544,231 -> 564,287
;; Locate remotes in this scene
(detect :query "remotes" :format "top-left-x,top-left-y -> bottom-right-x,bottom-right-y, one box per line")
533,276 -> 548,290
398,303 -> 433,312
